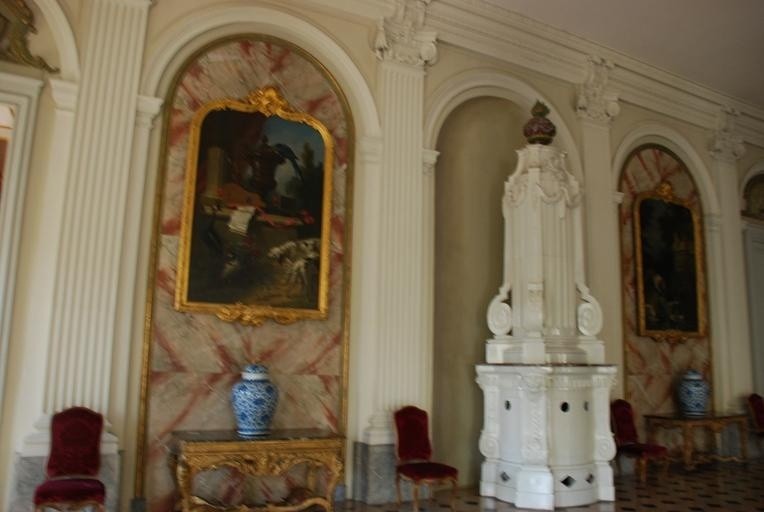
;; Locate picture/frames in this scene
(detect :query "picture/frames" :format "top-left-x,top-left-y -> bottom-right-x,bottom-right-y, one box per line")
172,86 -> 335,324
632,182 -> 710,343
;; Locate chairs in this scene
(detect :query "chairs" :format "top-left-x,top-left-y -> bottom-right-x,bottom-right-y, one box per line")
746,392 -> 764,440
31,407 -> 106,512
393,405 -> 458,512
610,399 -> 668,485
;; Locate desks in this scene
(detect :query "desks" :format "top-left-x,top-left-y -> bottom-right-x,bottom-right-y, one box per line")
643,411 -> 750,469
168,427 -> 347,512
